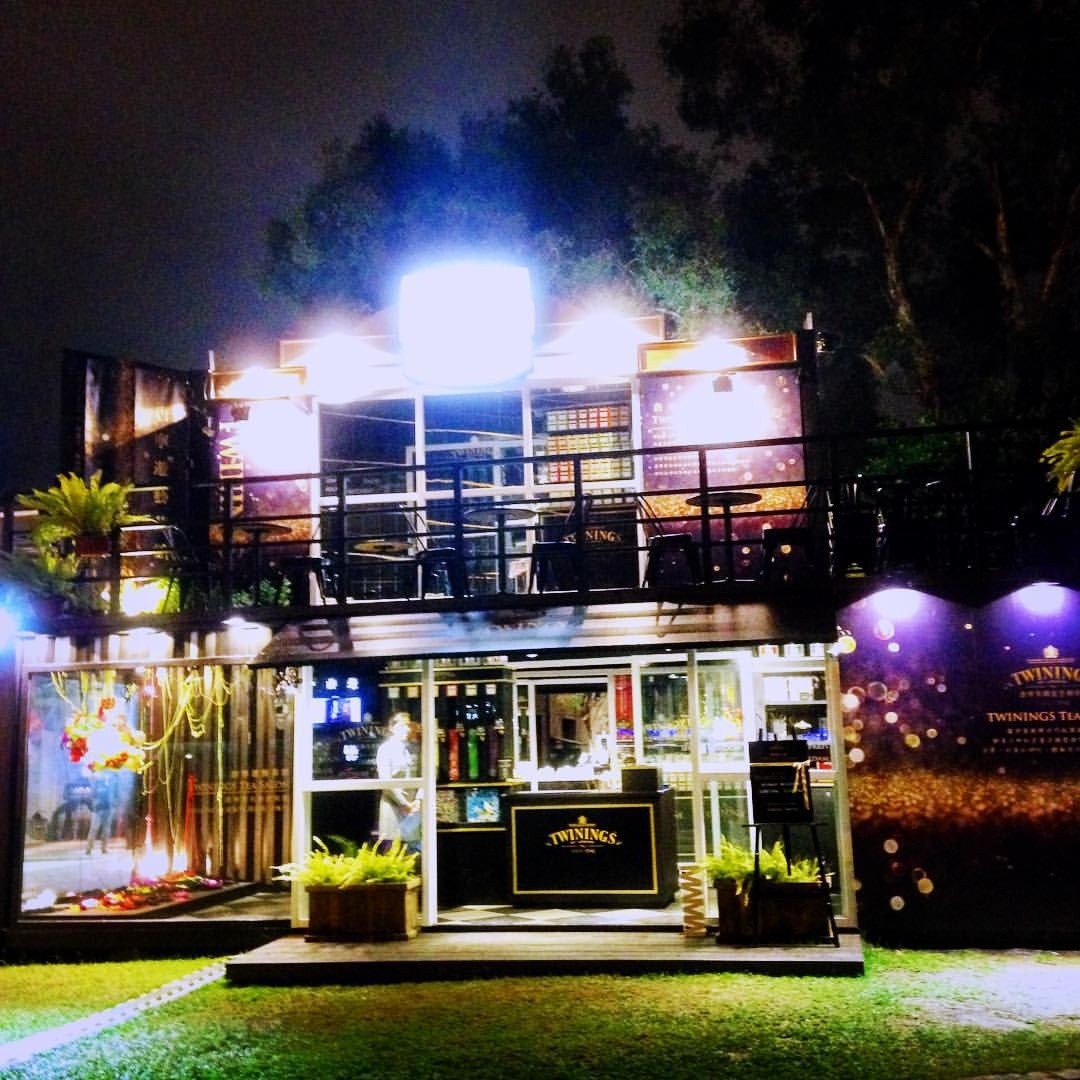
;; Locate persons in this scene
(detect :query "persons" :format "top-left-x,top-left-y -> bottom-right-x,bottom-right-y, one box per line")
81,699 -> 145,856
376,712 -> 421,921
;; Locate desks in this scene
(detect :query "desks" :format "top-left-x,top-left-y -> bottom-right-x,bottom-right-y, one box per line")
686,492 -> 762,581
218,523 -> 293,608
463,508 -> 537,595
354,542 -> 413,597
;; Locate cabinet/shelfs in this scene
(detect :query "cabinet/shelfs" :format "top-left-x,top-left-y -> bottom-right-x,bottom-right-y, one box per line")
436,782 -> 524,833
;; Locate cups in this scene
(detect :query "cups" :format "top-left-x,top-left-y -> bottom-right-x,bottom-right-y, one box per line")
769,716 -> 787,739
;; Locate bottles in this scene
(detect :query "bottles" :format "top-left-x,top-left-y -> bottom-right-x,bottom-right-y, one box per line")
449,730 -> 499,780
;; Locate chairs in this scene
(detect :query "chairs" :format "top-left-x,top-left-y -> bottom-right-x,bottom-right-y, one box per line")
755,485 -> 818,581
528,492 -> 593,595
399,504 -> 470,599
161,525 -> 220,614
274,522 -> 336,610
634,493 -> 702,587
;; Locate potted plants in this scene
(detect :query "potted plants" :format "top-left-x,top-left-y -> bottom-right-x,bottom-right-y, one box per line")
269,835 -> 422,943
702,834 -> 832,946
15,470 -> 157,557
